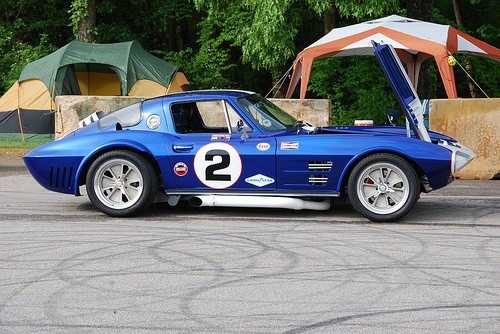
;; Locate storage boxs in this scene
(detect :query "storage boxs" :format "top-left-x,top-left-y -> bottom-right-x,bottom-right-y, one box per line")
354,119 -> 373,126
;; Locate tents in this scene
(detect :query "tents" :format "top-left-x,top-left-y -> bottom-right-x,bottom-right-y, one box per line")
0,38 -> 194,133
283,13 -> 500,126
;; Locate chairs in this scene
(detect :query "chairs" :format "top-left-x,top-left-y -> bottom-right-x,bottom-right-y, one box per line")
385,107 -> 403,126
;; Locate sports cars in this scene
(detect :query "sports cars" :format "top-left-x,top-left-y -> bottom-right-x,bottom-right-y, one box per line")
22,39 -> 477,222
